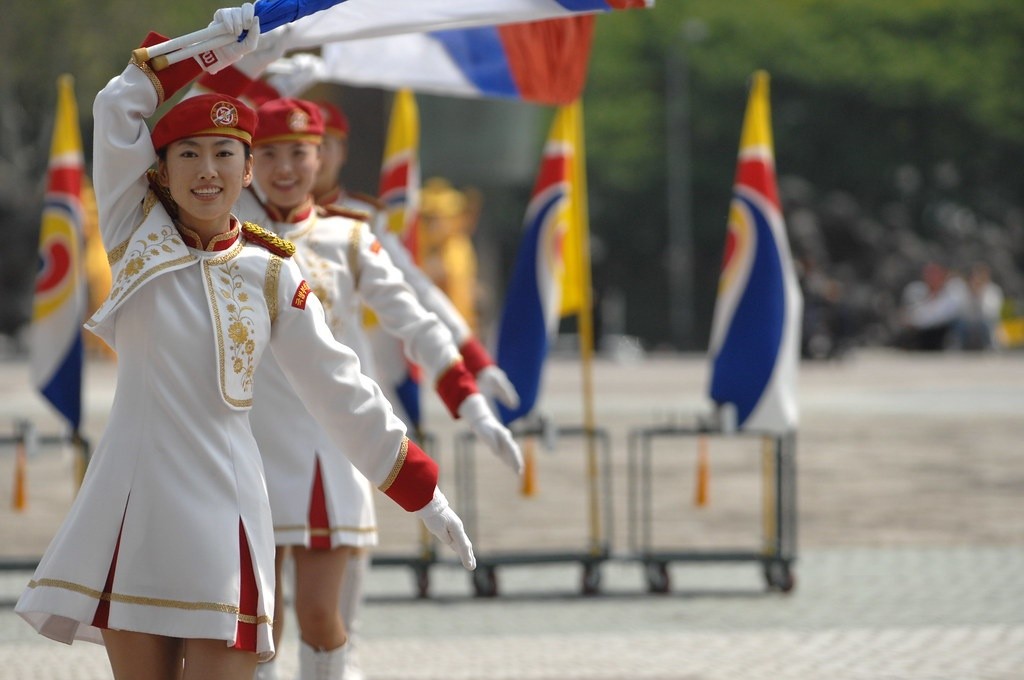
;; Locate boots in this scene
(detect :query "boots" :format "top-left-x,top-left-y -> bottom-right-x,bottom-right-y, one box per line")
338,590 -> 362,680
298,633 -> 348,680
254,659 -> 278,680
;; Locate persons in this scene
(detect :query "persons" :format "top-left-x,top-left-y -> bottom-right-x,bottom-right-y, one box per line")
417,179 -> 477,336
175,24 -> 520,680
894,261 -> 963,349
955,262 -> 1003,351
17,5 -> 477,680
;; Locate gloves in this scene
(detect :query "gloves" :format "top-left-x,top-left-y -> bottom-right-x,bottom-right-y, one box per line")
477,365 -> 520,409
191,3 -> 260,74
266,53 -> 327,97
231,23 -> 293,80
414,485 -> 476,571
458,392 -> 522,475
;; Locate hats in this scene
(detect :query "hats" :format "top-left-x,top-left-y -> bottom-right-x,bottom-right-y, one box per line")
314,101 -> 350,139
251,97 -> 324,147
151,93 -> 259,150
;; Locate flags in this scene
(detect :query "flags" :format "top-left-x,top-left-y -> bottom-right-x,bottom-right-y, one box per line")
496,100 -> 585,423
357,87 -> 419,429
698,72 -> 802,436
31,74 -> 114,436
244,0 -> 653,105
